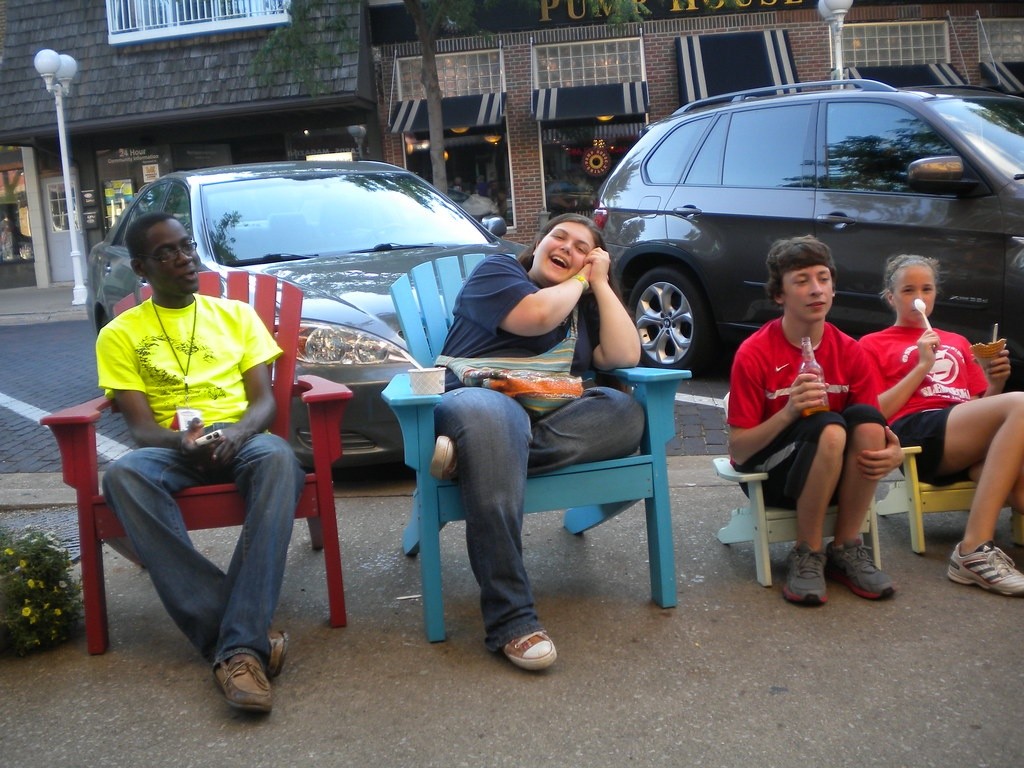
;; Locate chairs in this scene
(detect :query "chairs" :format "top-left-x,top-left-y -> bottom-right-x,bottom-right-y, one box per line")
380,253 -> 693,643
711,388 -> 882,588
876,444 -> 1013,554
38,268 -> 354,656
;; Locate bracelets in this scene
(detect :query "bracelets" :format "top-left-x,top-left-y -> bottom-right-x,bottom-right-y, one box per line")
572,275 -> 590,291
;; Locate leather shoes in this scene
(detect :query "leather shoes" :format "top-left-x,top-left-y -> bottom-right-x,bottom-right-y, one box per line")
213,653 -> 273,714
265,620 -> 290,678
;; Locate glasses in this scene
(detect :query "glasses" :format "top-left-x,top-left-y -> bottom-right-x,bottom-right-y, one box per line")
136,240 -> 197,263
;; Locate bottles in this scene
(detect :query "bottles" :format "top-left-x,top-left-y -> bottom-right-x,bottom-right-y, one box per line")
798,336 -> 829,417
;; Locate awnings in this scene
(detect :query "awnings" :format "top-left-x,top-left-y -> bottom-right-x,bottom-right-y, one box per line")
530,32 -> 649,120
844,11 -> 970,89
675,29 -> 802,108
976,10 -> 1024,95
388,39 -> 508,134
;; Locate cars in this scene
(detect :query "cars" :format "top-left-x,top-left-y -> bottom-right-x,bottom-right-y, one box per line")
86,160 -> 529,470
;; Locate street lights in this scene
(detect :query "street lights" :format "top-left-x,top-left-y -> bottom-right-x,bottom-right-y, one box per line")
35,51 -> 90,305
818,0 -> 854,90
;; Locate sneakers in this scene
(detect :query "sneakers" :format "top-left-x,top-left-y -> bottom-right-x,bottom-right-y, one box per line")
825,536 -> 897,598
782,540 -> 829,605
947,540 -> 1024,595
429,436 -> 458,483
504,629 -> 557,671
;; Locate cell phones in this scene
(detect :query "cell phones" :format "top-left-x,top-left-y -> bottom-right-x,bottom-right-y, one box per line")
195,429 -> 223,447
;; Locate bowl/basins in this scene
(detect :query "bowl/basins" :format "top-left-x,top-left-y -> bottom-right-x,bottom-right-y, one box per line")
408,368 -> 446,395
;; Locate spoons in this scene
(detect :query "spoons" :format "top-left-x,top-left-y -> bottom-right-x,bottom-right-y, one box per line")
914,299 -> 933,332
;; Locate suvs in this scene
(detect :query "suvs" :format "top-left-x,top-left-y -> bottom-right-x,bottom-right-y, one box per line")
592,80 -> 1024,392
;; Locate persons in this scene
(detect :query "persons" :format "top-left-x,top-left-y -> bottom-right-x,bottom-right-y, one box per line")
858,253 -> 1024,595
726,234 -> 906,605
429,213 -> 645,669
94,213 -> 305,714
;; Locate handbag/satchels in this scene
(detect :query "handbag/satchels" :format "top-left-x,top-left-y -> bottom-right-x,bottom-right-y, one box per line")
446,338 -> 583,419
